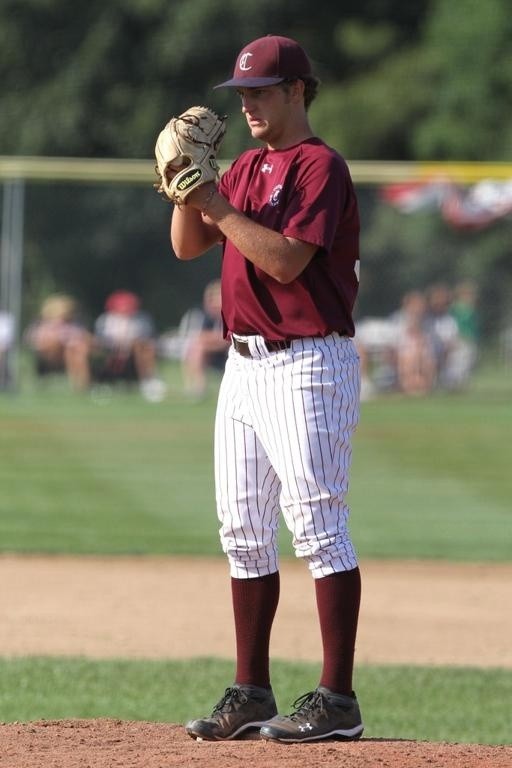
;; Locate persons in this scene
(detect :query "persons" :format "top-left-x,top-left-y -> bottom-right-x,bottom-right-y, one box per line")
154,32 -> 367,747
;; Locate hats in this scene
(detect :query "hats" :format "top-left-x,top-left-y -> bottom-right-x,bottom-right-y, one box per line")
211,31 -> 314,95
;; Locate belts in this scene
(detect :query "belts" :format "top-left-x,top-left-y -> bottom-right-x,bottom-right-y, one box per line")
231,337 -> 295,358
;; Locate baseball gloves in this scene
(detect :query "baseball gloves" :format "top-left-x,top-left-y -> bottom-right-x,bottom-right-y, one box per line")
154,105 -> 228,206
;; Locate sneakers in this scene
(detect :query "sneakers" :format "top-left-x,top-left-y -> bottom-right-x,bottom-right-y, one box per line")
184,682 -> 280,743
257,685 -> 366,744
